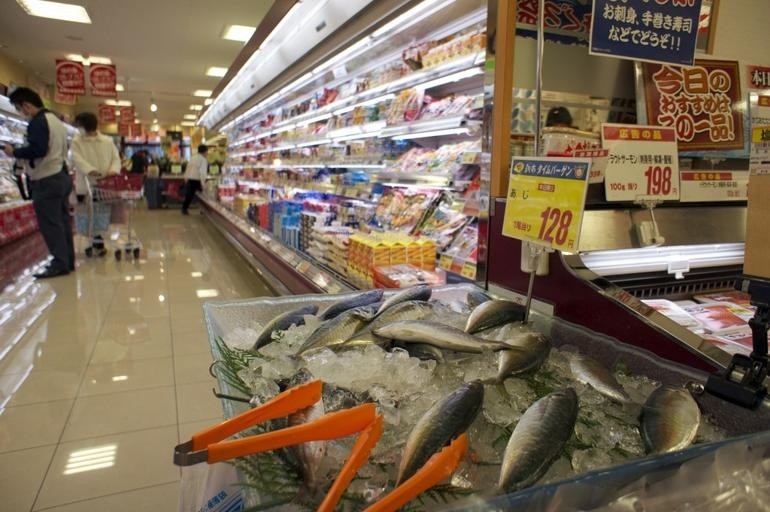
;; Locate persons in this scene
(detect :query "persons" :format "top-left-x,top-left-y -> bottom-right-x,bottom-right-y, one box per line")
84,252 -> 149,363
143,150 -> 154,166
28,275 -> 86,430
182,143 -> 209,215
68,111 -> 123,258
0,84 -> 75,280
542,106 -> 580,132
129,149 -> 146,172
179,215 -> 213,282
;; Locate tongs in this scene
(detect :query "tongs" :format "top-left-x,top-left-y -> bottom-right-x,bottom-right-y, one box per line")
315,413 -> 469,511
172,379 -> 376,466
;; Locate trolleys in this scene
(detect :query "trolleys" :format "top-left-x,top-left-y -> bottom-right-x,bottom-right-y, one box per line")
85,171 -> 143,261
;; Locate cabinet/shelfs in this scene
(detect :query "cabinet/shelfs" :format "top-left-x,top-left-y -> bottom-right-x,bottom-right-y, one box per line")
1,91 -> 83,290
198,0 -> 494,296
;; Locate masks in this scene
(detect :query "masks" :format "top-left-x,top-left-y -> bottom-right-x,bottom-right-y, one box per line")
78,125 -> 87,135
18,108 -> 33,121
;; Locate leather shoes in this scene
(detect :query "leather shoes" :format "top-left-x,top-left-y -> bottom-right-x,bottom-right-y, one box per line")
32,260 -> 76,280
